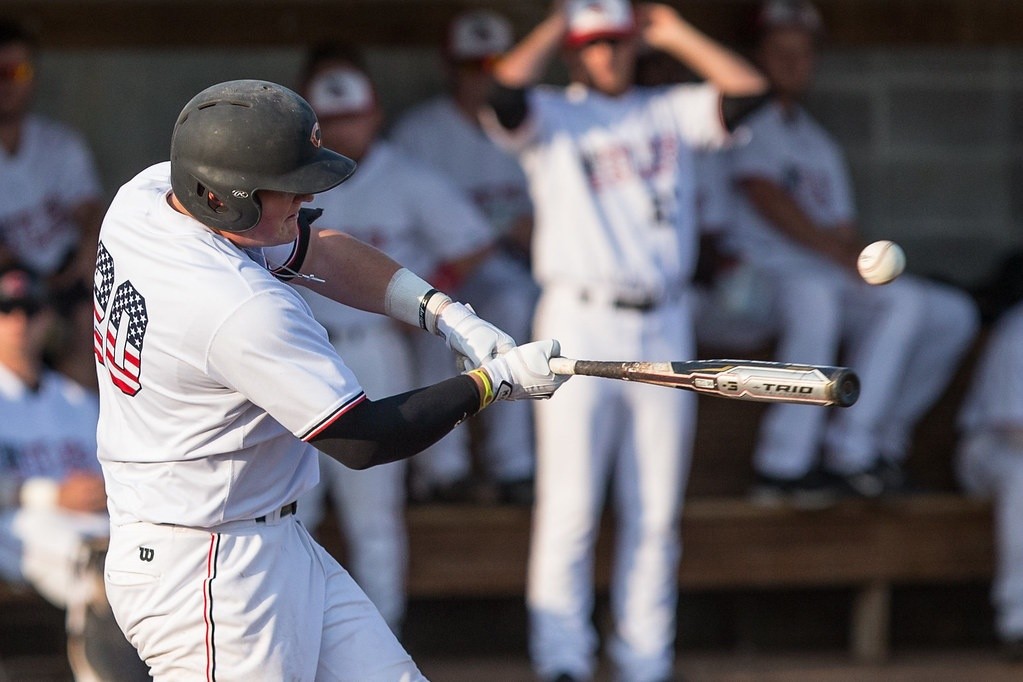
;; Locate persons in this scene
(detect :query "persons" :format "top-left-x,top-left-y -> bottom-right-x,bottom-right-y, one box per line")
273,0 -> 975,679
952,309 -> 1022,661
95,80 -> 571,682
0,32 -> 119,682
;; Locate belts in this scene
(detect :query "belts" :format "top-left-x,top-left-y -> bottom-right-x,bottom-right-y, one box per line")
256,500 -> 297,523
582,287 -> 655,311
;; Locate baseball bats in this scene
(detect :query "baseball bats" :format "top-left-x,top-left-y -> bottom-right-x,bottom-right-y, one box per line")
547,354 -> 864,407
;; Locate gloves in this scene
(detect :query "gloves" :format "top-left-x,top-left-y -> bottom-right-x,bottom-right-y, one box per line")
482,339 -> 572,399
437,301 -> 516,372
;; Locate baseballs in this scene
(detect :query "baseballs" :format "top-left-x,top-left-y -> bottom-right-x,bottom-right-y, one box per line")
857,240 -> 906,286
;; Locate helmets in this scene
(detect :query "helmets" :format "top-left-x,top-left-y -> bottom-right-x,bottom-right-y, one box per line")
171,80 -> 357,232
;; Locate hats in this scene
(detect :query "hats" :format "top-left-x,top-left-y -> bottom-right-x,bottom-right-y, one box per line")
560,0 -> 637,47
306,66 -> 372,115
448,11 -> 513,61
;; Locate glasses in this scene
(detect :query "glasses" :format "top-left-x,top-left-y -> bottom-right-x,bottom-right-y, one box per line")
0,290 -> 50,316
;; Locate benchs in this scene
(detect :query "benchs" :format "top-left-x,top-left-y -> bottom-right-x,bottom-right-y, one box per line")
318,349 -> 993,663
